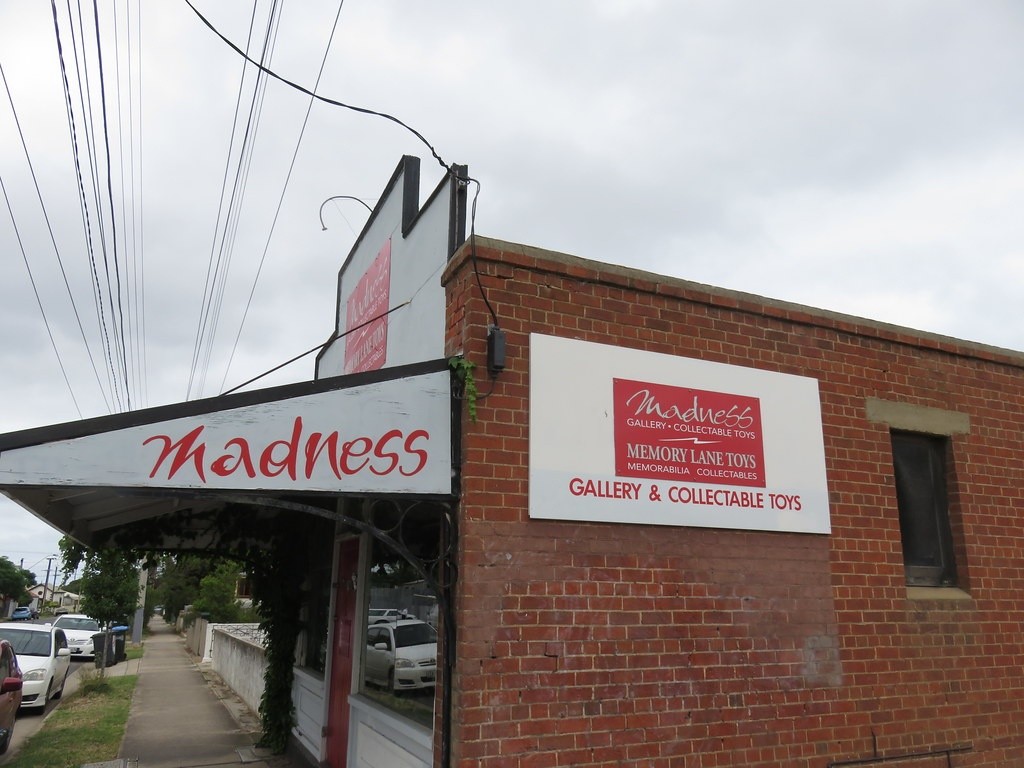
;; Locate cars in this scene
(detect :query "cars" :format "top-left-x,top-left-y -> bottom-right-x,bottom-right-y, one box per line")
364,608 -> 437,697
0,623 -> 72,722
11,606 -> 32,621
27,607 -> 39,620
45,613 -> 107,661
0,638 -> 23,754
55,607 -> 69,616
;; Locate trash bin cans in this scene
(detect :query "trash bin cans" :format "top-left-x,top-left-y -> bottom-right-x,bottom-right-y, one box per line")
90,631 -> 117,669
112,624 -> 129,661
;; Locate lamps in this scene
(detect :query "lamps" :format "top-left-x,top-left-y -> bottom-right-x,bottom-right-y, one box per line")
319,195 -> 374,231
